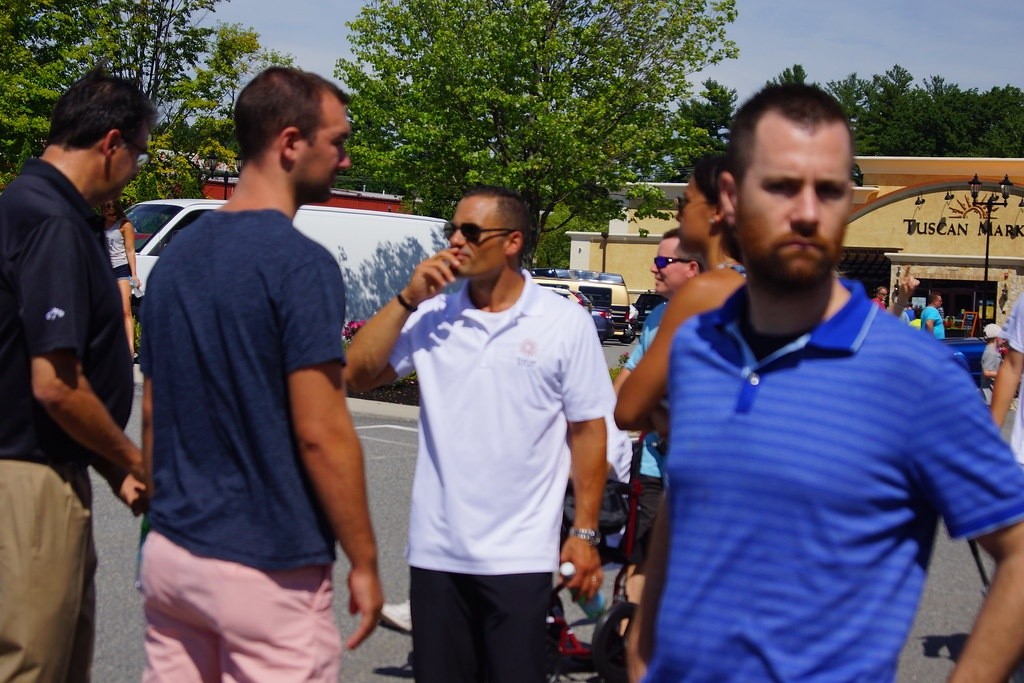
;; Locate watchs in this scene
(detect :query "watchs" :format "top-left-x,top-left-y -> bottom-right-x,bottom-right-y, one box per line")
570,528 -> 601,544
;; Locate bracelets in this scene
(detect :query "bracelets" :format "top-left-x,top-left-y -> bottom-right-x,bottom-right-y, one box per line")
396,293 -> 417,312
894,297 -> 909,309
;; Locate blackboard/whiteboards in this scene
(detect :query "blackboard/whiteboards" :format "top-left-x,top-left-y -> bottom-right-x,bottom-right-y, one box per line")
961,312 -> 980,337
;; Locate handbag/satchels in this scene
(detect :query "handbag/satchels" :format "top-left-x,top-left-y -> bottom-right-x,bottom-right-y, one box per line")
562,479 -> 657,552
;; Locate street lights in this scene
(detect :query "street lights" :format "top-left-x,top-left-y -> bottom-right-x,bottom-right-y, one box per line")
207,153 -> 228,200
967,172 -> 1013,332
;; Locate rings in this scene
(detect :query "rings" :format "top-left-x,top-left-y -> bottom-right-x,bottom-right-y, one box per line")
592,577 -> 601,581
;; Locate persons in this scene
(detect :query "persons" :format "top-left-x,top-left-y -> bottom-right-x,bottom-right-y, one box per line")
981,323 -> 1006,381
346,184 -> 617,683
886,275 -> 920,323
871,286 -> 888,309
641,83 -> 1024,683
137,67 -> 384,683
0,65 -> 160,683
616,230 -> 705,573
920,291 -> 945,339
615,150 -> 748,683
101,199 -> 141,362
904,301 -> 923,328
991,289 -> 1024,470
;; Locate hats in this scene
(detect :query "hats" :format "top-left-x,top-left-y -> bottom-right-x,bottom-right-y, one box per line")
984,323 -> 1011,339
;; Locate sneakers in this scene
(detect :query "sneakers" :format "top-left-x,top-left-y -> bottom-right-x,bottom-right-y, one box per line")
375,603 -> 415,633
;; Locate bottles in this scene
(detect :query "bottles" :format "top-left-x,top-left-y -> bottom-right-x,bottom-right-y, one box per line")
134,514 -> 149,594
559,561 -> 607,619
128,276 -> 143,298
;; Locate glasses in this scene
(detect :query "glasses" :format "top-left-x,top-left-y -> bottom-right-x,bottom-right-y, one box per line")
678,196 -> 704,211
117,129 -> 152,168
879,291 -> 888,298
444,222 -> 513,241
654,257 -> 703,270
101,205 -> 114,210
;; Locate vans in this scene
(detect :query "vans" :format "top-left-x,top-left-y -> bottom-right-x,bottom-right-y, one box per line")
529,268 -> 630,337
106,198 -> 455,327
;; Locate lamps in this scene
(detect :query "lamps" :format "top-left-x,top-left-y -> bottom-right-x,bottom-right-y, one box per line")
1018,197 -> 1024,207
990,184 -> 999,202
944,185 -> 954,201
894,279 -> 899,296
1001,283 -> 1008,300
914,191 -> 926,206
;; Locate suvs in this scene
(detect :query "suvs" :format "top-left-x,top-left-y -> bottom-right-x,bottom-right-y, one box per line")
619,293 -> 668,344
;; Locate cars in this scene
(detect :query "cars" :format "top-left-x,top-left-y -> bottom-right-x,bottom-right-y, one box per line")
544,287 -> 616,343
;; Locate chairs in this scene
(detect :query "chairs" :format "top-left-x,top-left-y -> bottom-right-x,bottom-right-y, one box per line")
954,352 -> 995,406
